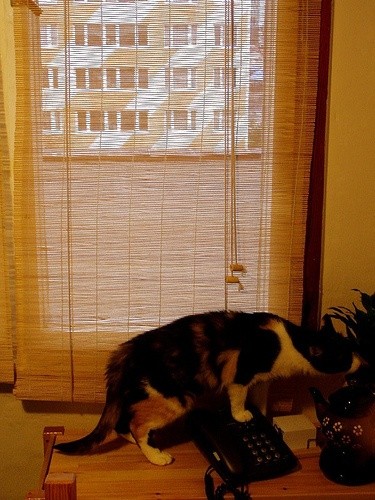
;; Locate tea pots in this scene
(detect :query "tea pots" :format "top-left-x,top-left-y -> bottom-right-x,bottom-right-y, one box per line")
308,372 -> 375,486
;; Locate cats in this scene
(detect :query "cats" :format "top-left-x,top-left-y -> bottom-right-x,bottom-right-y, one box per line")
53,308 -> 361,466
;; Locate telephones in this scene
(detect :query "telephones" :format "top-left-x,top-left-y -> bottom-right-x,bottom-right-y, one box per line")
192,410 -> 302,500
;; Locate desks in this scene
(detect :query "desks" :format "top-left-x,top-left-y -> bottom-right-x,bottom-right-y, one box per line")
40,426 -> 375,500
